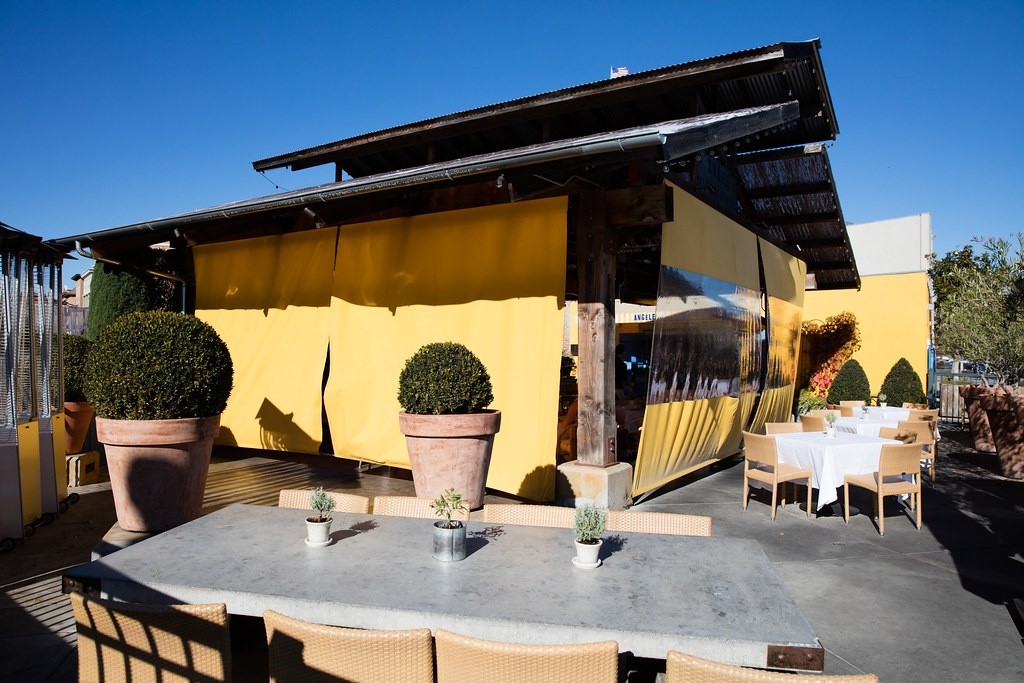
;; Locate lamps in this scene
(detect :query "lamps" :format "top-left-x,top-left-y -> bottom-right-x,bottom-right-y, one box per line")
494,173 -> 505,188
173,228 -> 182,238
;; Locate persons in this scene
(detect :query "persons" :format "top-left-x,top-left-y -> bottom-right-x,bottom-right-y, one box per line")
557,398 -> 578,455
615,344 -> 628,390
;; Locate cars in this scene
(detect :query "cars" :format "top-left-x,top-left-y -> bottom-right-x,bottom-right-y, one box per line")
935,354 -> 1000,374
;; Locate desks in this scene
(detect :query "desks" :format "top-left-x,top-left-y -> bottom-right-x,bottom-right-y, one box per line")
749,431 -> 905,516
832,417 -> 901,436
852,406 -> 909,420
60,505 -> 827,675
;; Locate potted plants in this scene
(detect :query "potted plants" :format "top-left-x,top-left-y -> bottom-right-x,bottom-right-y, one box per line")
932,236 -> 1024,478
879,394 -> 888,406
398,342 -> 501,516
429,489 -> 470,560
80,308 -> 234,529
932,240 -> 1013,452
571,507 -> 609,568
938,371 -> 972,423
862,402 -> 872,420
40,331 -> 96,453
305,487 -> 335,547
824,411 -> 837,439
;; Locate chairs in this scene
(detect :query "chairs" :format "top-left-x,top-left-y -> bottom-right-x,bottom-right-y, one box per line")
70,397 -> 939,683
